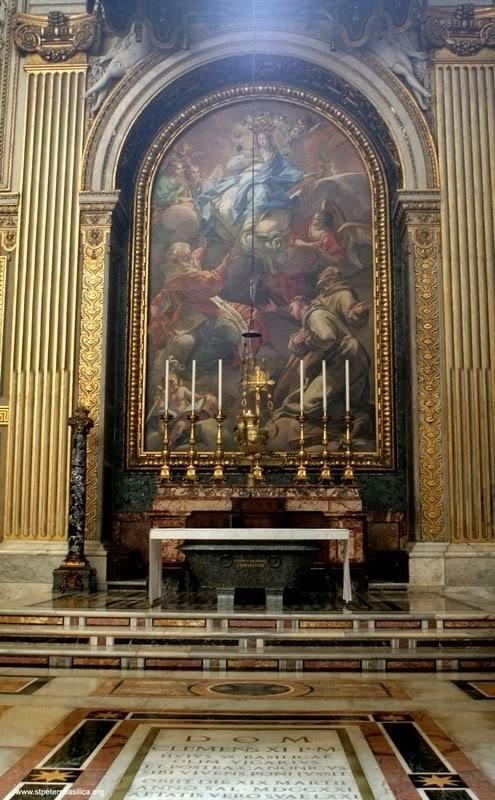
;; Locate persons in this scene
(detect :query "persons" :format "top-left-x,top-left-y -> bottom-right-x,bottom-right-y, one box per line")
360,15 -> 435,111
80,18 -> 153,118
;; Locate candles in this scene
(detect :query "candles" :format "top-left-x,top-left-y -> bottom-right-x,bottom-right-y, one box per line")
191,359 -> 196,410
165,359 -> 169,410
218,359 -> 222,410
345,360 -> 350,412
299,360 -> 304,412
322,359 -> 327,412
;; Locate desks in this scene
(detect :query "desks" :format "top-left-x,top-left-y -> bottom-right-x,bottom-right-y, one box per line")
148,527 -> 352,606
